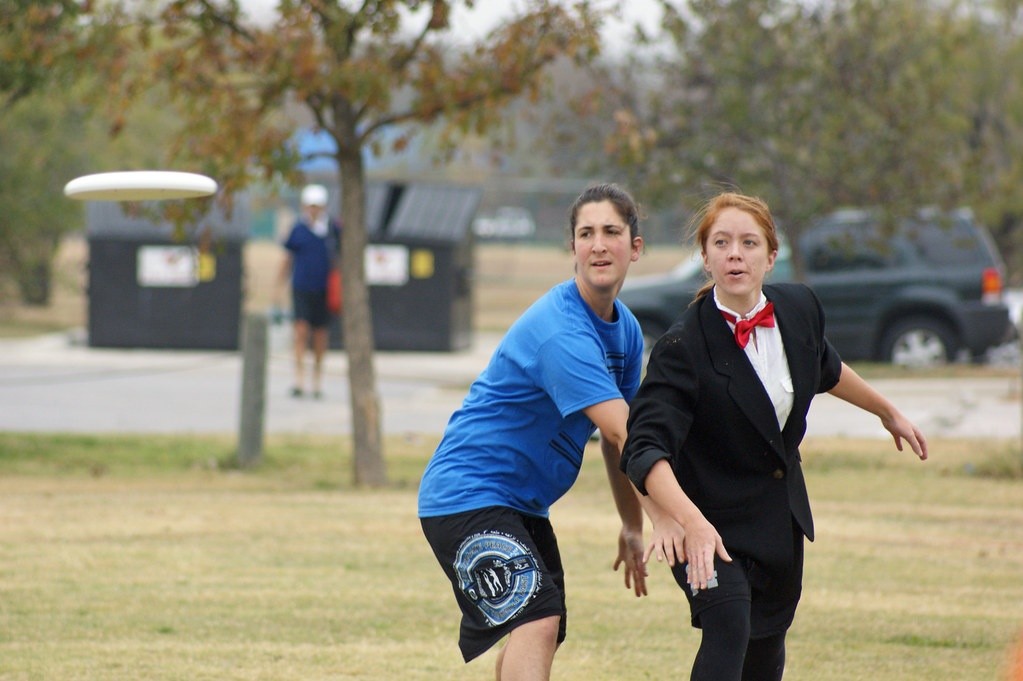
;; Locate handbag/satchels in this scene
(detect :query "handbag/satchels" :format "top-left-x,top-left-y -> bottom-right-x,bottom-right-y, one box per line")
327,271 -> 344,313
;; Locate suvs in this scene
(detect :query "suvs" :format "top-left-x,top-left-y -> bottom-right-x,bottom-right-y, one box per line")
617,198 -> 1017,367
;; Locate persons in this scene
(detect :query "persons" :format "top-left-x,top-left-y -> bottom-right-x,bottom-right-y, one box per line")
414,184 -> 691,679
272,183 -> 348,405
621,192 -> 928,681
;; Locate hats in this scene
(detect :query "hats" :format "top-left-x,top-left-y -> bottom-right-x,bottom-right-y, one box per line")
301,186 -> 328,207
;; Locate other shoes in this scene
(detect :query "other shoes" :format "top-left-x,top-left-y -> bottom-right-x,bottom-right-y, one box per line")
293,387 -> 327,399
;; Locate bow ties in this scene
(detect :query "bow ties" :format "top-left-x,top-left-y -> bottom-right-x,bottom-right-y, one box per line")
718,300 -> 775,349
